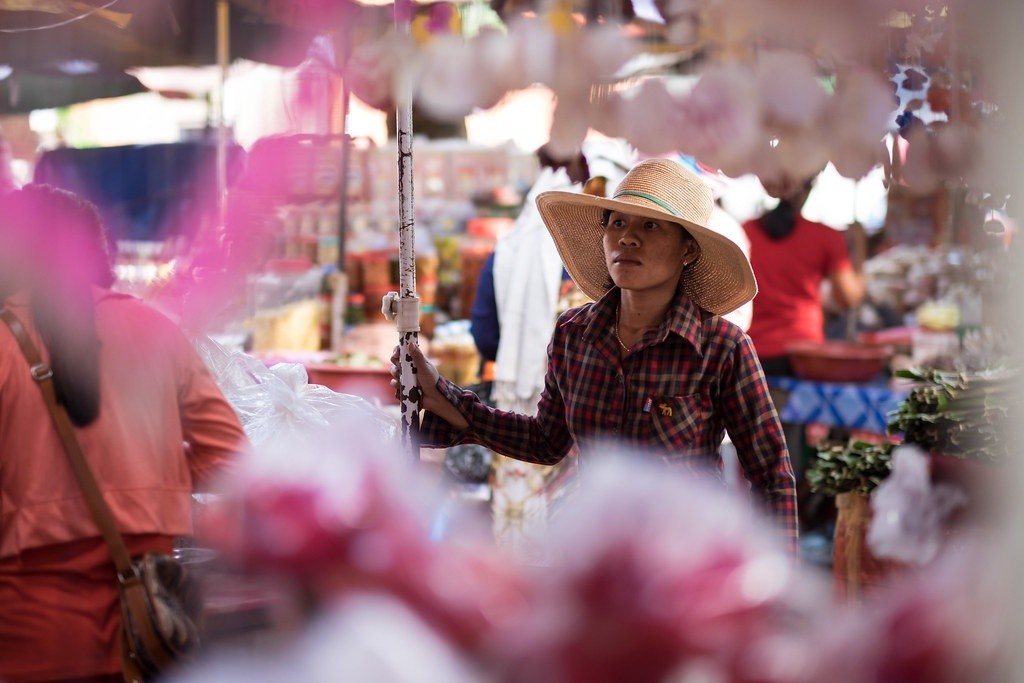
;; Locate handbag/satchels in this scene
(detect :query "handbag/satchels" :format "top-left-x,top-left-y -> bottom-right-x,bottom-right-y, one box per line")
117,549 -> 204,676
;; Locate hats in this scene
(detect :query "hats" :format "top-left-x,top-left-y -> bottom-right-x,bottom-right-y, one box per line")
533,158 -> 758,316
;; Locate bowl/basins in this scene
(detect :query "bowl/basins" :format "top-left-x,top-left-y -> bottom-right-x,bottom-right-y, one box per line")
173,548 -> 215,578
788,341 -> 894,382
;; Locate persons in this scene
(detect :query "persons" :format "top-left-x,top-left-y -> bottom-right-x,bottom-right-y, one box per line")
471,166 -> 596,552
388,156 -> 800,566
741,175 -> 863,379
1,181 -> 253,682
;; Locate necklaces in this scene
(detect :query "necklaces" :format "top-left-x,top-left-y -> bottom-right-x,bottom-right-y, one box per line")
616,306 -> 631,352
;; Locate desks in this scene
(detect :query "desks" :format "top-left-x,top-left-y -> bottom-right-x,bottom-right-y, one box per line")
765,375 -> 918,533
